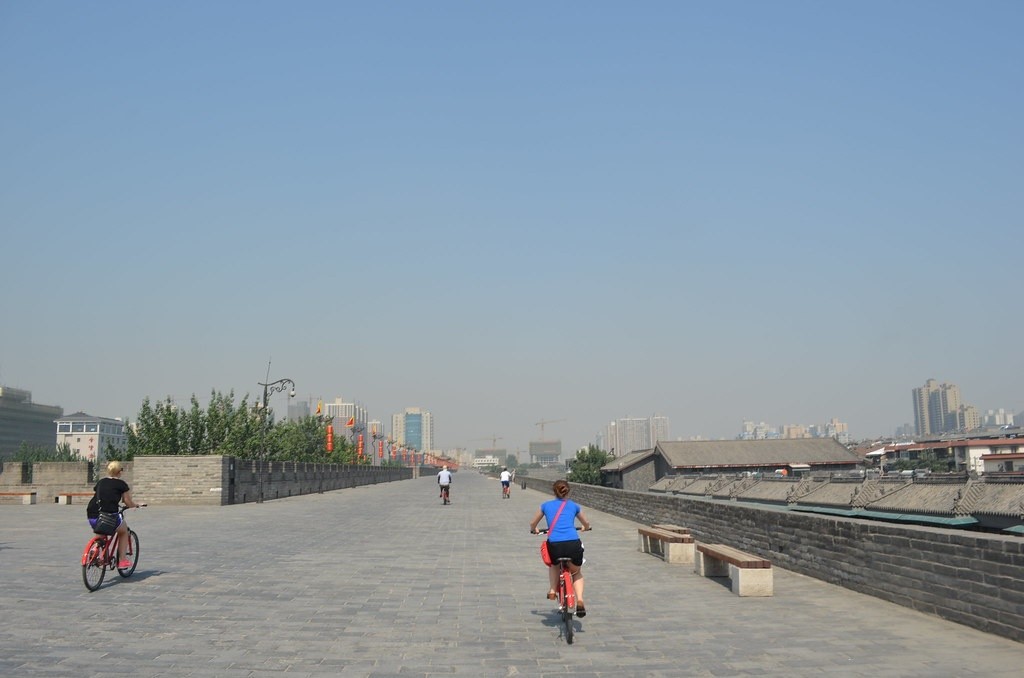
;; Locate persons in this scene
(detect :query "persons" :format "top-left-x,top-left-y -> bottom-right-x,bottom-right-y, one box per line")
86,461 -> 141,570
500,467 -> 511,497
511,468 -> 516,481
437,465 -> 452,503
530,480 -> 590,618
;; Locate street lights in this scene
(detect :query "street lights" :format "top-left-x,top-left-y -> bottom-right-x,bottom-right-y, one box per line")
258,378 -> 296,503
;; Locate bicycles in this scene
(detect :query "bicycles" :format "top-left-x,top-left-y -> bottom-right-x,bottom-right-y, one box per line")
502,483 -> 510,499
438,482 -> 452,505
81,504 -> 147,591
531,526 -> 594,644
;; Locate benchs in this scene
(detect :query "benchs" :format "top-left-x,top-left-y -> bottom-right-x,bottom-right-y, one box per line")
652,523 -> 691,537
0,490 -> 37,504
638,528 -> 696,564
695,542 -> 775,597
59,491 -> 95,505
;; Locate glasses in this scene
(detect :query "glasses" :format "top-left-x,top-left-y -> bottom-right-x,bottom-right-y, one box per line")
118,467 -> 123,473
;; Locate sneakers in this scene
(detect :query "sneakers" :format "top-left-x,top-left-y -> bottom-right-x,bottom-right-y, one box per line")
89,546 -> 98,560
576,601 -> 586,618
117,558 -> 133,568
546,592 -> 556,600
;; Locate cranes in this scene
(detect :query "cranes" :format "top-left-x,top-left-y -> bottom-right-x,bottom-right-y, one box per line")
535,417 -> 566,441
469,432 -> 501,447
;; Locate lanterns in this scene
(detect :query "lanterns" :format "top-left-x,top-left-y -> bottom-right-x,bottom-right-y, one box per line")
325,425 -> 444,467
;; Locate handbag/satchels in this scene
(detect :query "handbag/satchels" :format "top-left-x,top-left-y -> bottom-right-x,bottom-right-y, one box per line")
540,541 -> 552,567
93,511 -> 117,536
